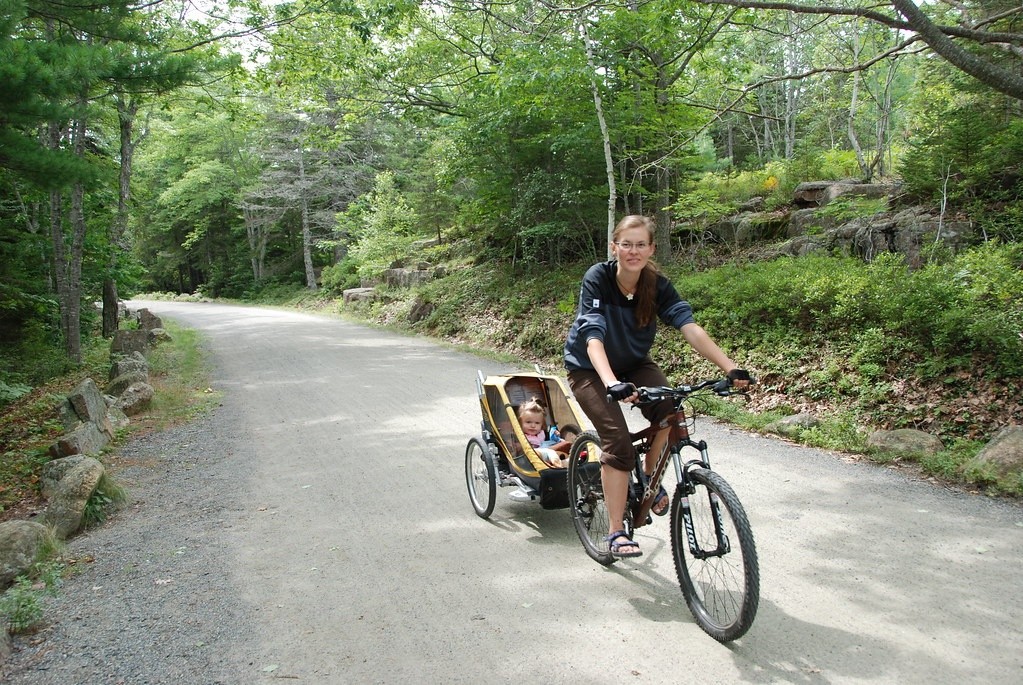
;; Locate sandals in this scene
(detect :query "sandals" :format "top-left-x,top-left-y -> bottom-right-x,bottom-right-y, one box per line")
603,530 -> 642,557
633,460 -> 669,516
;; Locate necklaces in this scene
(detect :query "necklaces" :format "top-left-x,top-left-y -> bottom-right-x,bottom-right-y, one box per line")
616,277 -> 635,300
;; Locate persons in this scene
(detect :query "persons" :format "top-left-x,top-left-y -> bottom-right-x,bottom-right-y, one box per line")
514,397 -> 586,469
562,215 -> 752,558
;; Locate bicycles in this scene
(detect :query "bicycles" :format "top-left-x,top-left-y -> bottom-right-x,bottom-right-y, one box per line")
566,378 -> 762,643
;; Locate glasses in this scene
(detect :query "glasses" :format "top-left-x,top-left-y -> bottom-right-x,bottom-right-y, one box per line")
615,241 -> 651,250
